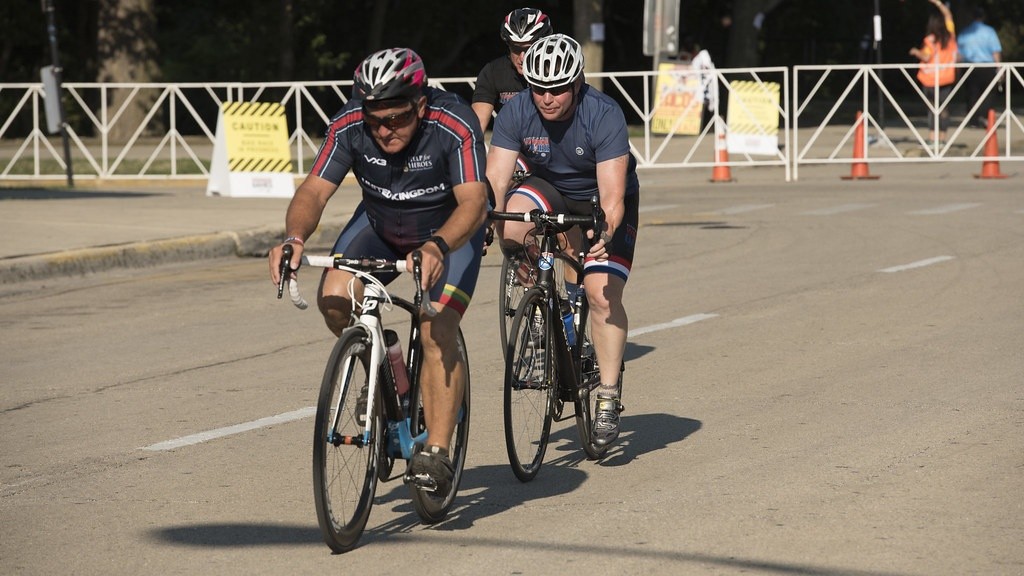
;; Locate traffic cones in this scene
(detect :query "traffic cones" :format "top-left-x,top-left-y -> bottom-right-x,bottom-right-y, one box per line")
840,111 -> 882,181
707,116 -> 739,183
974,108 -> 1010,179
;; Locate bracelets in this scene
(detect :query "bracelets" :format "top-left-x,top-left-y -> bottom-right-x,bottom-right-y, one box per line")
427,236 -> 449,255
936,1 -> 941,6
285,237 -> 303,243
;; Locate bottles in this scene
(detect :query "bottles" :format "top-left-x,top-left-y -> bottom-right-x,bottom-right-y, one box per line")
383,328 -> 410,396
560,303 -> 577,345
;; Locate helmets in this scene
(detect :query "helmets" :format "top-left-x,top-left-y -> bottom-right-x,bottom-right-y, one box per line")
500,7 -> 555,45
352,47 -> 426,102
520,32 -> 584,89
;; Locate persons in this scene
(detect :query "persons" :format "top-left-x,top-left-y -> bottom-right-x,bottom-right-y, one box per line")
485,33 -> 641,446
270,48 -> 495,496
471,8 -> 584,348
910,0 -> 957,150
957,9 -> 1002,129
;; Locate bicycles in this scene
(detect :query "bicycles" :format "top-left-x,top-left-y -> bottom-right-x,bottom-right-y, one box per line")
481,209 -> 626,483
276,243 -> 472,550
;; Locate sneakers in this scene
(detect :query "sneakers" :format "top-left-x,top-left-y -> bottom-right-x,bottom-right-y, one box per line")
413,445 -> 454,494
590,392 -> 625,446
521,313 -> 546,346
354,378 -> 368,427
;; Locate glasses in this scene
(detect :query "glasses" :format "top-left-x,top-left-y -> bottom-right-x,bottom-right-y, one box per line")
509,43 -> 531,57
531,85 -> 573,96
360,100 -> 417,129
